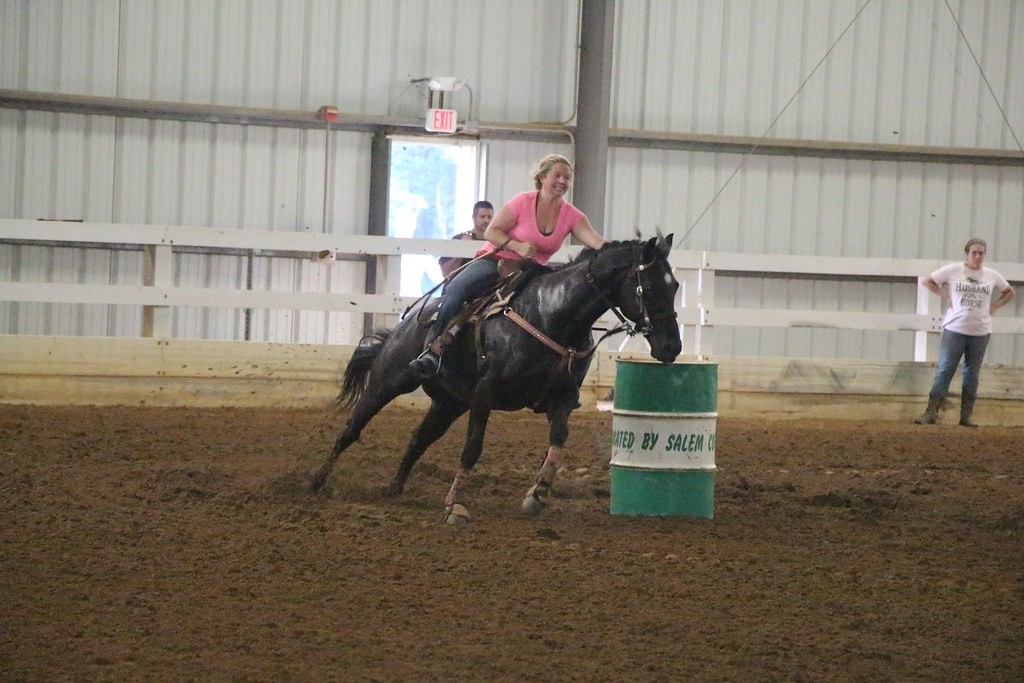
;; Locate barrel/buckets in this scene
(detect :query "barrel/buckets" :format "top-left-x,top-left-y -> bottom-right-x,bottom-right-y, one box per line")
609,358 -> 720,519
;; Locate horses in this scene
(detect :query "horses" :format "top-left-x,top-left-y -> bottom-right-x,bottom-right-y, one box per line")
305,225 -> 682,525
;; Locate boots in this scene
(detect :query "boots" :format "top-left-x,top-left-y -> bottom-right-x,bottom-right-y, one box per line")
959,398 -> 980,426
914,399 -> 942,424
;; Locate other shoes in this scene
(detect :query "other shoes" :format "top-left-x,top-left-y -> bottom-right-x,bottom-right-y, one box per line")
408,350 -> 440,375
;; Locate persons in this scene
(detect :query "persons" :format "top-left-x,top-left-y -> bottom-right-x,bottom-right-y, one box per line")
435,201 -> 496,300
914,237 -> 1017,429
405,152 -> 611,379
